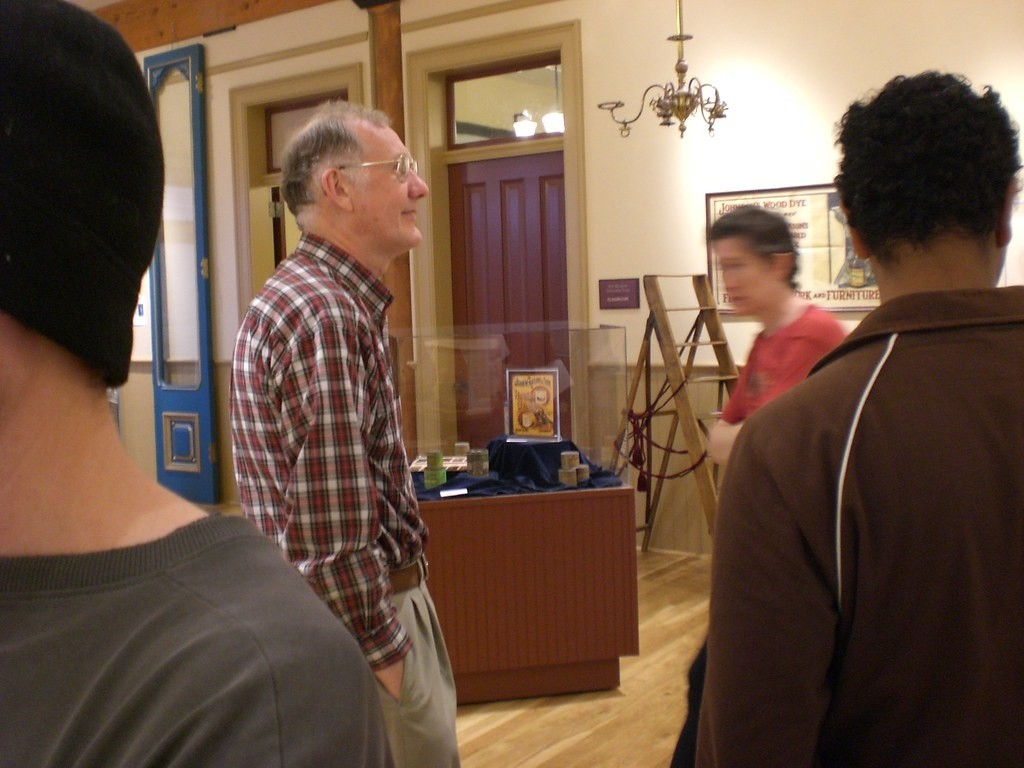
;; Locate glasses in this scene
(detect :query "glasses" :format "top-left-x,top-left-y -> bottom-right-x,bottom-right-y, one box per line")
333,154 -> 417,184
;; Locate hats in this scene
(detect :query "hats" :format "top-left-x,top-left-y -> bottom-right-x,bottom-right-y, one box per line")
0,0 -> 166,388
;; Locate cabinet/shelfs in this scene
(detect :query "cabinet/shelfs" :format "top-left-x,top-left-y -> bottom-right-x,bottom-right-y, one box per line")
391,450 -> 642,705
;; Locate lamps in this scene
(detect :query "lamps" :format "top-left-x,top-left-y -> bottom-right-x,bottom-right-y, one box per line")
592,0 -> 729,139
513,64 -> 565,138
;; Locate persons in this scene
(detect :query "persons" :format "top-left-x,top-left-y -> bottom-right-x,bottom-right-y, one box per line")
668,205 -> 849,767
696,70 -> 1024,768
229,100 -> 461,768
0,1 -> 395,768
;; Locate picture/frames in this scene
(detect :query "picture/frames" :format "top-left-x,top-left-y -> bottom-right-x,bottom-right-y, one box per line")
705,181 -> 880,314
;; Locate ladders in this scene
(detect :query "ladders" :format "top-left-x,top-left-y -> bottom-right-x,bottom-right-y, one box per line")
608,274 -> 741,553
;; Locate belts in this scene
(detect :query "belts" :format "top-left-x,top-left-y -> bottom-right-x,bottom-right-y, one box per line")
388,554 -> 429,596
